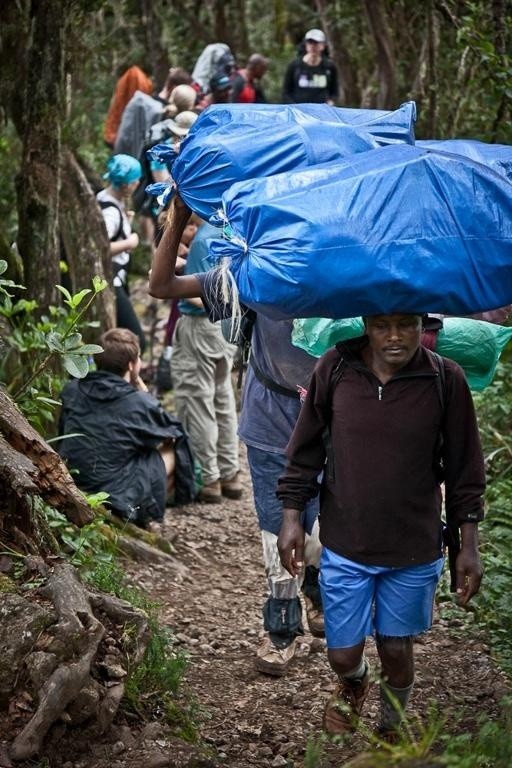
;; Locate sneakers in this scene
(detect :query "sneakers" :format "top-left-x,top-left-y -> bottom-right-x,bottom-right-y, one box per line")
255,632 -> 297,675
321,660 -> 374,736
373,724 -> 404,756
224,474 -> 244,499
302,596 -> 325,638
197,479 -> 223,503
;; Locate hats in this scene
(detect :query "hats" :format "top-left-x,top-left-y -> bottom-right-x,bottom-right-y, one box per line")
166,111 -> 198,136
306,28 -> 325,41
211,72 -> 233,91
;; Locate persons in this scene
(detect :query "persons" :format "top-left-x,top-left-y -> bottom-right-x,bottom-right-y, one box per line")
277,28 -> 338,106
275,311 -> 485,744
149,194 -> 325,676
54,28 -> 265,528
